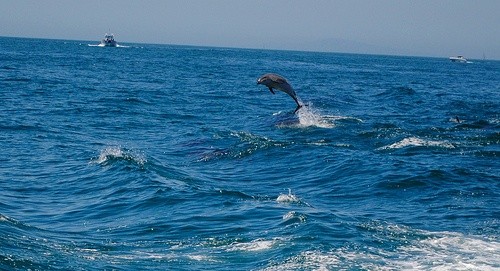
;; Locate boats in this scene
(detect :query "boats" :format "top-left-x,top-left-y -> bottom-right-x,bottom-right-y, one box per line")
104,33 -> 116,47
449,55 -> 467,63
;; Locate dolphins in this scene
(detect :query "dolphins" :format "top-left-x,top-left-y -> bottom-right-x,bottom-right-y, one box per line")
257,73 -> 308,116
